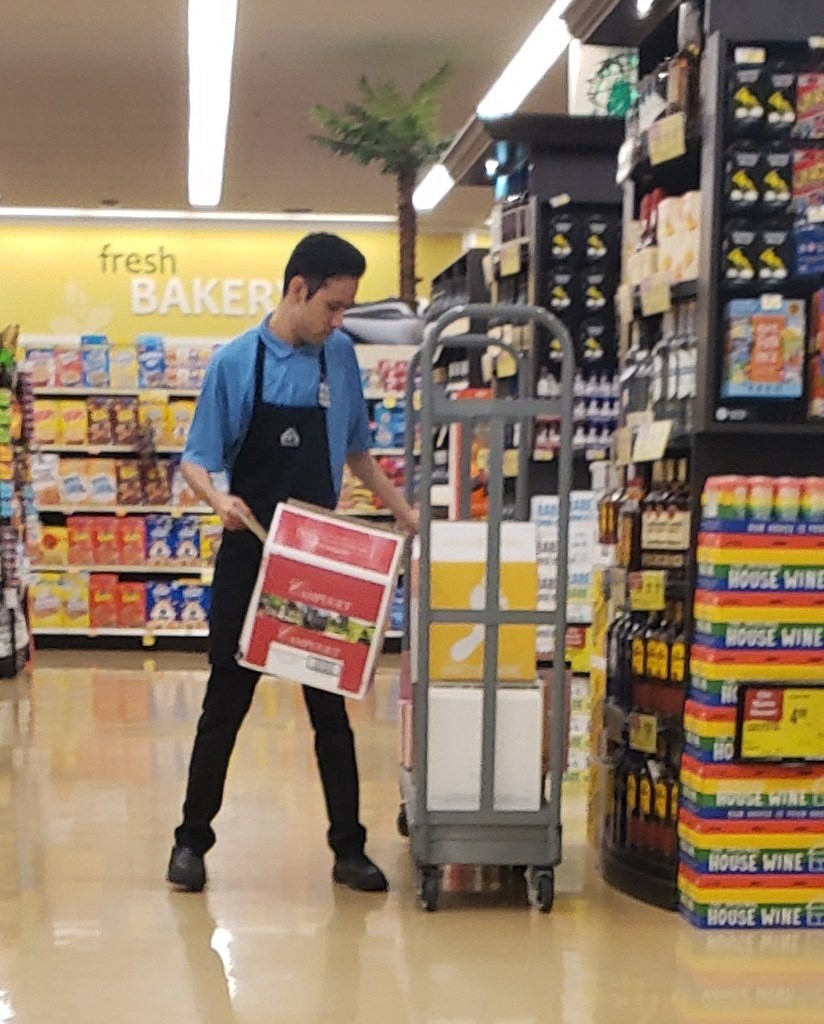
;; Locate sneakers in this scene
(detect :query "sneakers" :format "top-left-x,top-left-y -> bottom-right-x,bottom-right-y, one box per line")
169,845 -> 206,889
334,855 -> 388,893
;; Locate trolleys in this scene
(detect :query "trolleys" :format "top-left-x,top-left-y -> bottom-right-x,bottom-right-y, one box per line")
398,304 -> 574,912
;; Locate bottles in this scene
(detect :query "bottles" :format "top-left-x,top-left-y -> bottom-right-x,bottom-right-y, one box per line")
598,188 -> 700,862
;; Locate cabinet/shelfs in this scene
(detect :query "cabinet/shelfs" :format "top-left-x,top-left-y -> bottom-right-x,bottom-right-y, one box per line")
0,0 -> 824,931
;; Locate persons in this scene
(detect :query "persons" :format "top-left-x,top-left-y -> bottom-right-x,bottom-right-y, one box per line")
166,231 -> 422,893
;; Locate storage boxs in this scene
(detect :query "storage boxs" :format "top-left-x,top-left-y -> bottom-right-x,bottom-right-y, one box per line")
176,581 -> 211,623
120,516 -> 145,564
199,514 -> 223,567
89,574 -> 118,628
67,516 -> 94,564
116,581 -> 147,627
59,459 -> 88,505
59,572 -> 91,630
173,516 -> 200,566
722,296 -> 804,399
427,679 -> 546,812
146,580 -> 180,623
145,514 -> 173,566
27,573 -> 60,629
88,459 -> 115,505
141,458 -> 170,506
94,516 -> 119,565
410,522 -> 538,680
230,497 -> 409,699
116,459 -> 141,505
